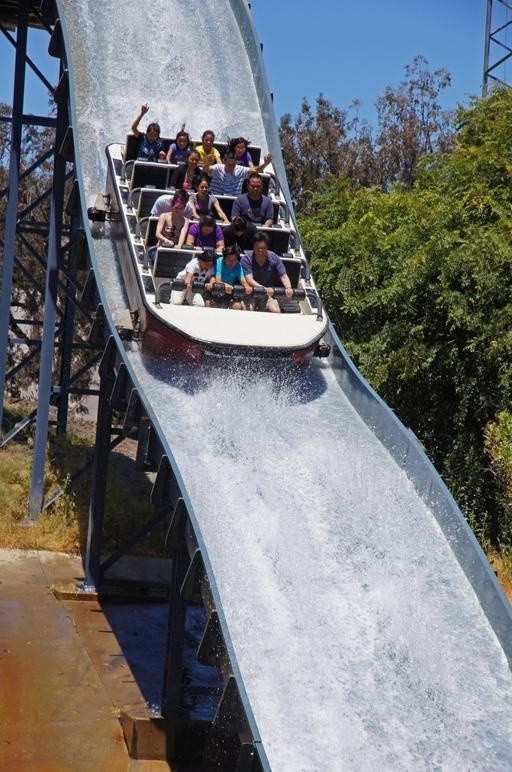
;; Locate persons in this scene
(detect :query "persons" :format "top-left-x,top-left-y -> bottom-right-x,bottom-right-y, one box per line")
131,100 -> 295,252
239,230 -> 293,313
203,245 -> 254,311
169,248 -> 218,308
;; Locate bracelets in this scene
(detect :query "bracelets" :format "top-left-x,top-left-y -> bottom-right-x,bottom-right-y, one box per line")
185,282 -> 192,286
285,287 -> 293,290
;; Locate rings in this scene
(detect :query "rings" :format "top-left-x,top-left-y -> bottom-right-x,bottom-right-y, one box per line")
287,291 -> 291,295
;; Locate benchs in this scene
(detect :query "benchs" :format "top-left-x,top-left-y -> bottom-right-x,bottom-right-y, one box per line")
126,132 -> 307,292
